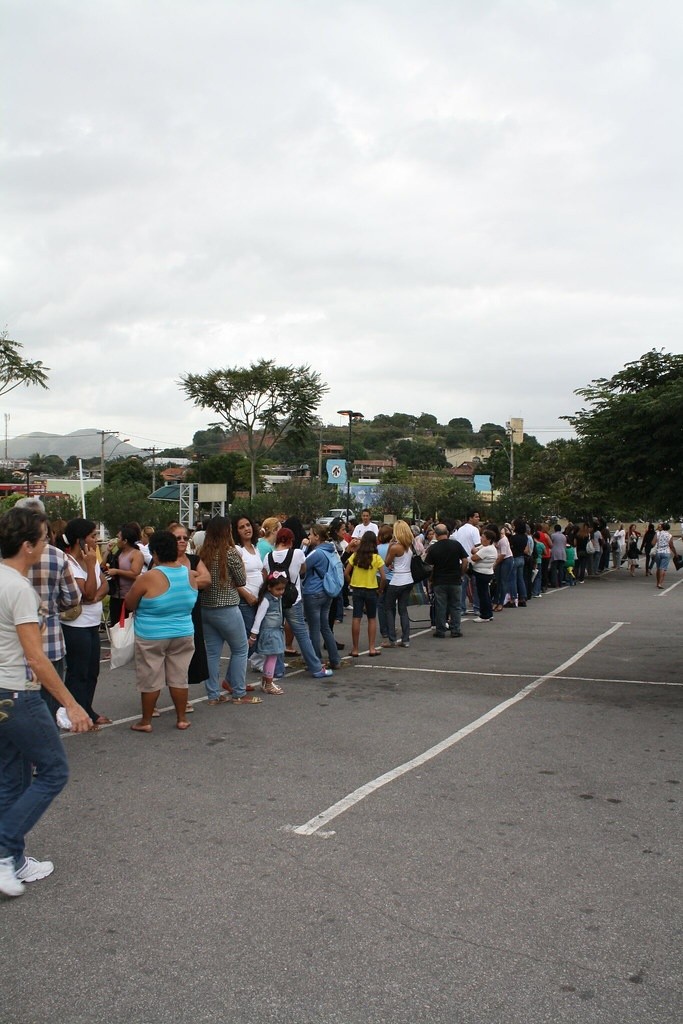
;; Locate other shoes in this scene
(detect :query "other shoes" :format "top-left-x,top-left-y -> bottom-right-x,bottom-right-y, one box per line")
285,650 -> 301,656
178,720 -> 190,729
433,632 -> 445,638
336,642 -> 345,650
451,632 -> 463,637
131,724 -> 153,732
473,616 -> 493,622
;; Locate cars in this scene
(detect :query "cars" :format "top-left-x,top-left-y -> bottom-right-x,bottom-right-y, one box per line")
316,509 -> 356,529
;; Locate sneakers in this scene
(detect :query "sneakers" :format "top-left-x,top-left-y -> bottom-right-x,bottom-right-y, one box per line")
0,855 -> 25,896
16,856 -> 54,882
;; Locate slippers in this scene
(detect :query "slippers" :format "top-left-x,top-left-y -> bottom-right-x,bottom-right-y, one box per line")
370,649 -> 381,656
349,650 -> 358,657
313,668 -> 334,677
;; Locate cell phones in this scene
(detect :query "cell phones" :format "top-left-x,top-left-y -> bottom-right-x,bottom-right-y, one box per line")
80,540 -> 86,554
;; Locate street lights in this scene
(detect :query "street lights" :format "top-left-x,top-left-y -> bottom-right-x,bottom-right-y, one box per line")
336,410 -> 364,531
99,438 -> 130,543
493,439 -> 516,508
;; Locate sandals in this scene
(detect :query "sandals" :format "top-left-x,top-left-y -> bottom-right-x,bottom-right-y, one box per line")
210,695 -> 230,705
261,680 -> 284,694
231,696 -> 263,704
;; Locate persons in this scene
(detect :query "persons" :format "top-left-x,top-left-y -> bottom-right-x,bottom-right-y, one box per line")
612,524 -> 625,570
455,511 -> 611,622
625,525 -> 640,576
330,509 -> 469,648
188,517 -> 334,707
0,508 -> 94,897
651,523 -> 678,589
102,522 -> 155,628
125,531 -> 200,732
14,498 -> 83,726
48,518 -> 114,729
640,523 -> 656,576
344,531 -> 387,656
149,523 -> 209,716
298,524 -> 341,669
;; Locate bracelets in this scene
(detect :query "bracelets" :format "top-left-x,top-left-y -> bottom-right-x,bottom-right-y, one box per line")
247,597 -> 252,603
250,636 -> 256,641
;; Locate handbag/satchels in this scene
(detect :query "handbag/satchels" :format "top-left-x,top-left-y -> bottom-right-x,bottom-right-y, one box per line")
650,543 -> 657,557
586,540 -> 595,554
411,545 -> 432,583
611,541 -> 618,551
110,601 -> 135,670
673,554 -> 683,570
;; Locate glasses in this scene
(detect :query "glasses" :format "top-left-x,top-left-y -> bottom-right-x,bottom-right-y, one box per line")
176,536 -> 189,541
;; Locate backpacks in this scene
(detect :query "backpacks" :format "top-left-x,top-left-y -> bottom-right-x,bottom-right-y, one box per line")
313,548 -> 344,598
268,549 -> 298,608
